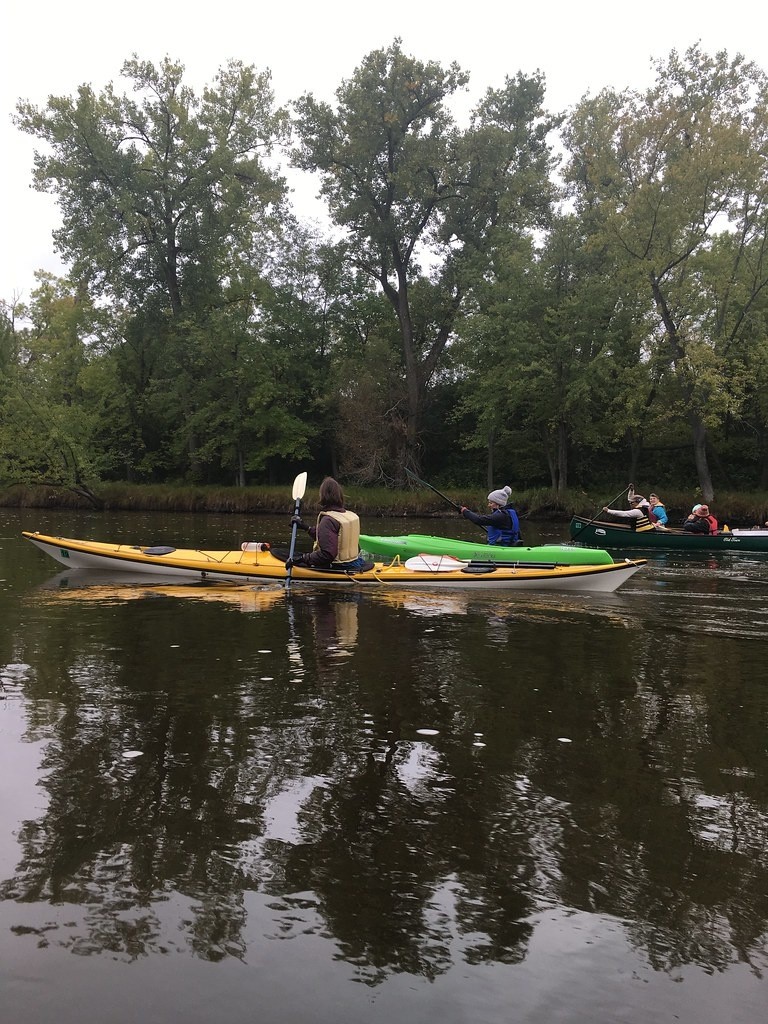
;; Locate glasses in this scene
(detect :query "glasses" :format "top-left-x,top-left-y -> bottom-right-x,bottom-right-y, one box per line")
630,502 -> 639,504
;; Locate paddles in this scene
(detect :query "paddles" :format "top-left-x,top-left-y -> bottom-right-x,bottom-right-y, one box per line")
401,463 -> 491,534
566,484 -> 634,546
405,552 -> 570,573
281,471 -> 309,593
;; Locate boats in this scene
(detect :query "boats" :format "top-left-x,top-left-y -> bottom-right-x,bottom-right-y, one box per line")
357,534 -> 615,565
17,530 -> 651,589
567,515 -> 768,552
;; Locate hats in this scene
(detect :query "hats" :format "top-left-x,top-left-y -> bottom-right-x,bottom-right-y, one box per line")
628,495 -> 644,503
487,486 -> 512,507
692,503 -> 702,513
694,505 -> 709,517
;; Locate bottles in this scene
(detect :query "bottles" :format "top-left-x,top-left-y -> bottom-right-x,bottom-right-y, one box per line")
241,542 -> 270,552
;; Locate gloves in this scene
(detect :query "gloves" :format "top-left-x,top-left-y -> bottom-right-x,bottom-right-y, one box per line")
629,483 -> 634,490
457,505 -> 464,514
289,515 -> 310,531
285,554 -> 304,570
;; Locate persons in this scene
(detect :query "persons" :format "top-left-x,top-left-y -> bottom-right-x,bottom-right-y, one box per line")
462,486 -> 523,547
684,504 -> 718,535
603,484 -> 668,534
284,476 -> 366,571
